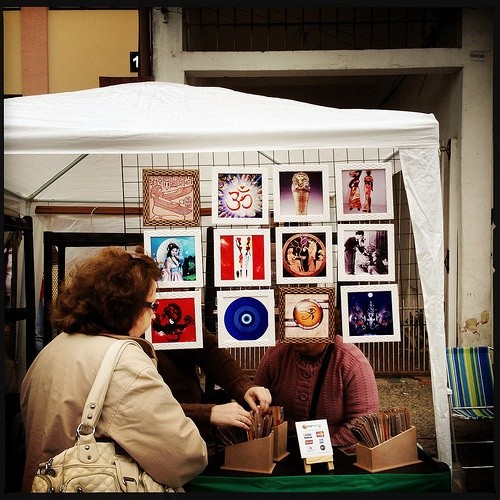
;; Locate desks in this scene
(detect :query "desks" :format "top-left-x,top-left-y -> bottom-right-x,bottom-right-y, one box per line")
183,447 -> 452,494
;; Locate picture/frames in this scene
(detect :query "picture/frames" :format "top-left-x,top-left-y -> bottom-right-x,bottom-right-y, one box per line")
144,163 -> 402,350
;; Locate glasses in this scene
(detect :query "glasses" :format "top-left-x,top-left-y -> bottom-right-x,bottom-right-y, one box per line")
143,302 -> 159,316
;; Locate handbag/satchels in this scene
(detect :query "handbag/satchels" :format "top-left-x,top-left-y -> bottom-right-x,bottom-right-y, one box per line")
31,339 -> 185,493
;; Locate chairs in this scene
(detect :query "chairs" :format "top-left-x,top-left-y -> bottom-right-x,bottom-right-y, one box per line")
446,347 -> 496,469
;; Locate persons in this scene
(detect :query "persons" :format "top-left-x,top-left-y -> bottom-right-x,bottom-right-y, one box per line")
344,231 -> 387,276
348,170 -> 373,212
18,249 -> 209,494
160,243 -> 184,281
134,244 -> 273,457
256,332 -> 380,448
